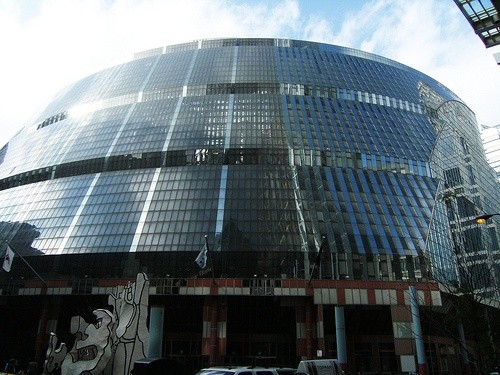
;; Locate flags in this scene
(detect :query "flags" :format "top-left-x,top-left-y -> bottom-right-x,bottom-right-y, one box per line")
194,242 -> 209,269
314,241 -> 324,266
1,245 -> 15,272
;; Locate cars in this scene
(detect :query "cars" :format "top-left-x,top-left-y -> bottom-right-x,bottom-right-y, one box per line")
196,365 -> 295,375
